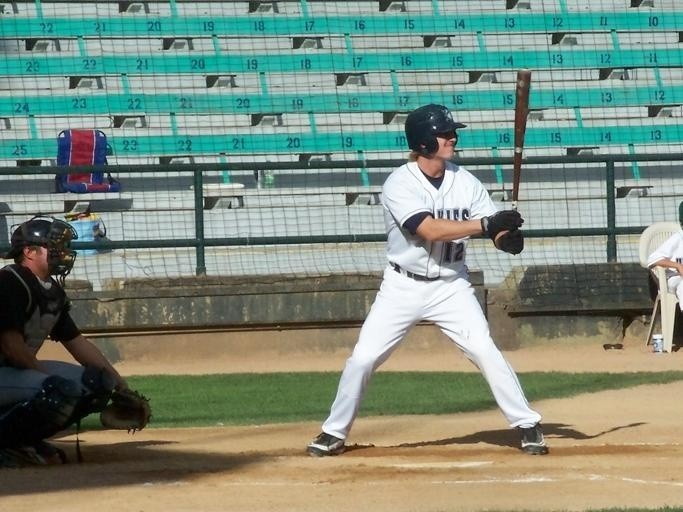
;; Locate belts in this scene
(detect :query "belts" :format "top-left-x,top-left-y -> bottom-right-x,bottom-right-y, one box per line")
390,262 -> 439,281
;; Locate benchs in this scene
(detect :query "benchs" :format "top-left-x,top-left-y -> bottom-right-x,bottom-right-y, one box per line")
0,0 -> 681,219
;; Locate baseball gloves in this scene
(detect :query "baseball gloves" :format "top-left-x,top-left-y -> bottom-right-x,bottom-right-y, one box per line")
100,386 -> 149,430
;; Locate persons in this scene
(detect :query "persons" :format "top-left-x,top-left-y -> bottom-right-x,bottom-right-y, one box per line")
304,104 -> 551,459
647,199 -> 683,315
0,214 -> 152,468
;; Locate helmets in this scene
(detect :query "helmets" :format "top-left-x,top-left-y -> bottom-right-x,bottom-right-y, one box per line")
11,218 -> 78,275
405,104 -> 466,155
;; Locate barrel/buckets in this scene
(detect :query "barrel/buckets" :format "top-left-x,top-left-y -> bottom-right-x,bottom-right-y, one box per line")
67,216 -> 108,258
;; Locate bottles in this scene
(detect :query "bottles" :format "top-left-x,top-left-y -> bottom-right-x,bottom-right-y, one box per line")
263,159 -> 274,189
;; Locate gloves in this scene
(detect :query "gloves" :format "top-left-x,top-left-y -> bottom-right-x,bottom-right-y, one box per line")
480,210 -> 524,253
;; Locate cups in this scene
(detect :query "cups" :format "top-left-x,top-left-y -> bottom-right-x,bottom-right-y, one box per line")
652,333 -> 664,354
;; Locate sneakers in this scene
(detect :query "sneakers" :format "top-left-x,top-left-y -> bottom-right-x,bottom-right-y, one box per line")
307,432 -> 345,457
519,423 -> 548,455
0,439 -> 72,466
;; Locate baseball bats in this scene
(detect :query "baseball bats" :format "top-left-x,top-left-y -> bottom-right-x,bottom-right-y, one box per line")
511,70 -> 531,211
